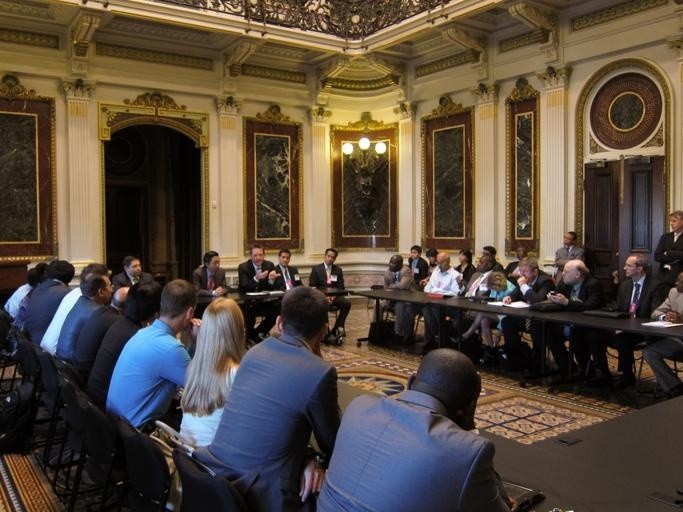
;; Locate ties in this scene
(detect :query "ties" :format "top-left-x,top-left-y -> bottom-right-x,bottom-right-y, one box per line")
208,275 -> 213,289
464,275 -> 483,297
632,283 -> 639,305
284,270 -> 289,280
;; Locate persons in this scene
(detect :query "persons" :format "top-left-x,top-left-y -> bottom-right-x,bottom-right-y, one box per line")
314,347 -> 519,511
179,297 -> 248,457
384,245 -> 557,379
652,210 -> 683,286
236,243 -> 282,345
575,253 -> 666,392
554,231 -> 586,286
3,256 -> 163,412
190,285 -> 341,511
105,279 -> 201,436
193,252 -> 227,319
270,248 -> 303,290
642,274 -> 683,399
547,260 -> 607,392
308,248 -> 350,336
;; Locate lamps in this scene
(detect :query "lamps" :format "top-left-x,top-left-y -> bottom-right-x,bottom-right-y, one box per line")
340,136 -> 388,197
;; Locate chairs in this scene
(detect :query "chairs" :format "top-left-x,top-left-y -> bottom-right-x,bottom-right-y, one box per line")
0,308 -> 251,511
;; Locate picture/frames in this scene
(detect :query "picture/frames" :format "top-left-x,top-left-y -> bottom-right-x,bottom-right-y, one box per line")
328,110 -> 400,254
0,71 -> 61,268
420,92 -> 476,255
240,102 -> 306,257
503,75 -> 541,260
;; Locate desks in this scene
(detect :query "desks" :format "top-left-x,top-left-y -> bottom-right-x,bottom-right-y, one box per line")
353,287 -> 683,396
195,287 -> 349,347
310,379 -> 682,512
